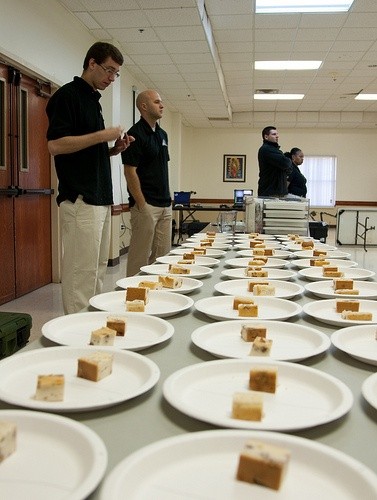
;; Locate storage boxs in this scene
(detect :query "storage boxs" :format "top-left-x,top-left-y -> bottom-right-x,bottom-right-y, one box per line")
0,311 -> 32,360
174,192 -> 190,203
183,220 -> 199,232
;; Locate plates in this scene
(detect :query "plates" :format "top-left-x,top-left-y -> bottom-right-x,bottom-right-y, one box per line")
0,232 -> 377,500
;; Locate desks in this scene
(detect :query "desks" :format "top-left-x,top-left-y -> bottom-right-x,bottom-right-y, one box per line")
0,205 -> 377,500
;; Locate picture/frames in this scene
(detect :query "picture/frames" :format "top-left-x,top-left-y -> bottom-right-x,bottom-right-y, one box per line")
223,154 -> 246,182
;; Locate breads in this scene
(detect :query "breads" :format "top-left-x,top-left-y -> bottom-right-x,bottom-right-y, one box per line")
237,440 -> 292,490
36,375 -> 65,402
90,232 -> 373,354
249,366 -> 278,393
0,422 -> 17,461
77,353 -> 114,380
232,392 -> 264,420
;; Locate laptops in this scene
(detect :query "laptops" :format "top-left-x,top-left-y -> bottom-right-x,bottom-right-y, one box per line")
173,192 -> 191,207
233,190 -> 253,208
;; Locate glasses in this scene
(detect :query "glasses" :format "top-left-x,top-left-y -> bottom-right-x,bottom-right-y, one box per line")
99,63 -> 120,78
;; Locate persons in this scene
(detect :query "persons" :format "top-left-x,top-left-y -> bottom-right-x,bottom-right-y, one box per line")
258,127 -> 306,198
44,42 -> 135,316
121,88 -> 173,276
